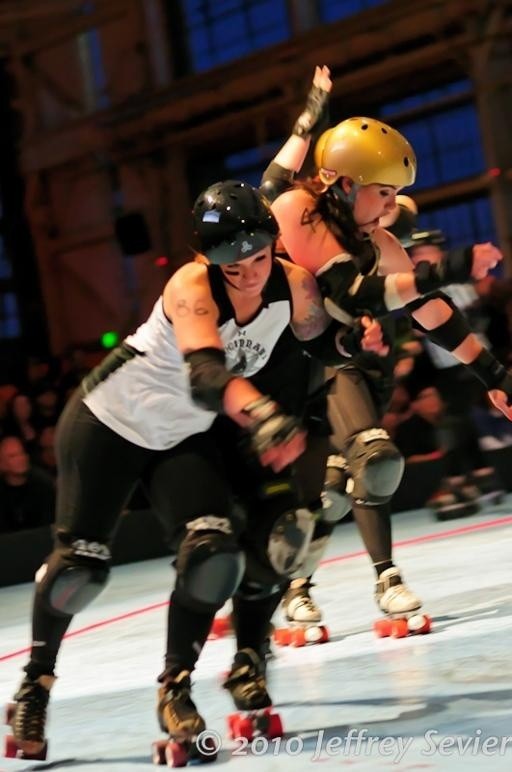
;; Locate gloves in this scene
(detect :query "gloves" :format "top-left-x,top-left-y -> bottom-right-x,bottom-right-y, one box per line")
343,314 -> 393,359
292,82 -> 330,136
414,242 -> 477,294
471,345 -> 511,412
241,397 -> 305,452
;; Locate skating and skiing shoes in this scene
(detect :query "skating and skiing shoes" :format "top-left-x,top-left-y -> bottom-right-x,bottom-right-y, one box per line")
274,577 -> 331,647
225,658 -> 284,743
4,672 -> 48,761
370,567 -> 431,638
151,681 -> 217,768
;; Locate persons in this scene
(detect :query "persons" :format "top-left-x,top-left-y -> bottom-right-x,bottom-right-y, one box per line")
260,61 -> 423,622
11,181 -> 331,756
0,276 -> 512,522
226,115 -> 512,708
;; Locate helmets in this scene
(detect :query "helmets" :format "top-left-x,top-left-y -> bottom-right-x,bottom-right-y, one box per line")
188,179 -> 279,264
315,116 -> 416,189
